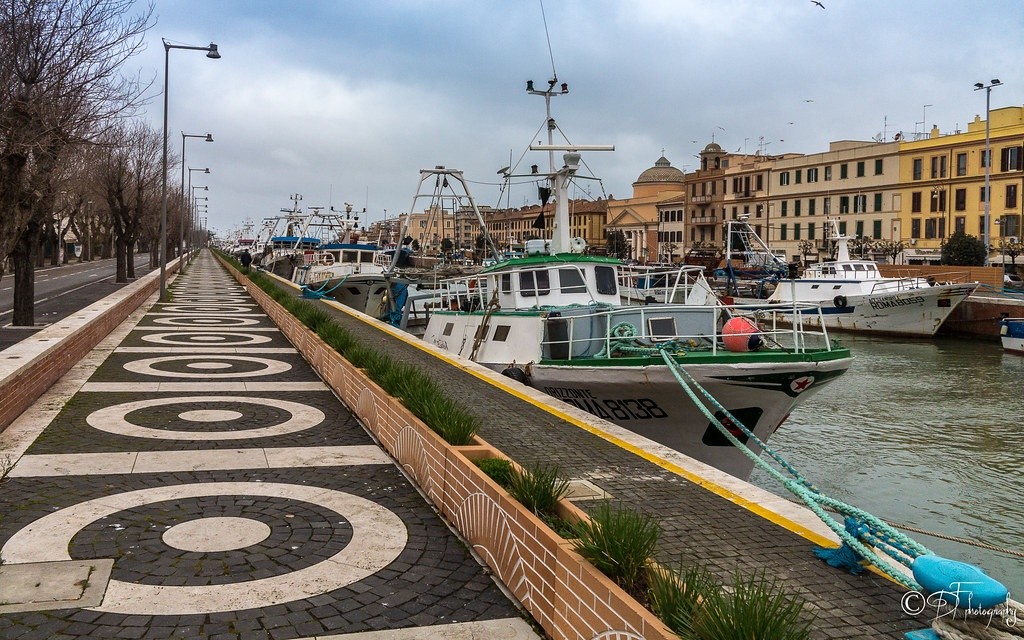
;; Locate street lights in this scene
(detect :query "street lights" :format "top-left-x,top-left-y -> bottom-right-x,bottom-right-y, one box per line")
187,167 -> 210,266
180,131 -> 215,274
160,36 -> 221,302
57,191 -> 67,267
745,137 -> 749,155
973,79 -> 1004,267
88,201 -> 92,262
923,104 -> 932,139
192,185 -> 209,261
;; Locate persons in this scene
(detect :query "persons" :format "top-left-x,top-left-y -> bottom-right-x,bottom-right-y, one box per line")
241,249 -> 252,275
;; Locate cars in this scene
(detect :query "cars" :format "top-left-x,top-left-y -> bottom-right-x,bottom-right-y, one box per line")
1004,273 -> 1023,288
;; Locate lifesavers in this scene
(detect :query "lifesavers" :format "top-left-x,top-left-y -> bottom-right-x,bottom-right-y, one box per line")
310,241 -> 317,248
286,258 -> 298,269
372,253 -> 388,266
320,253 -> 336,267
833,295 -> 847,309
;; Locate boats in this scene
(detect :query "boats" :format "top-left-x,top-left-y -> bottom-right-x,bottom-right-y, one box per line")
714,168 -> 980,339
213,0 -> 856,483
999,318 -> 1024,356
